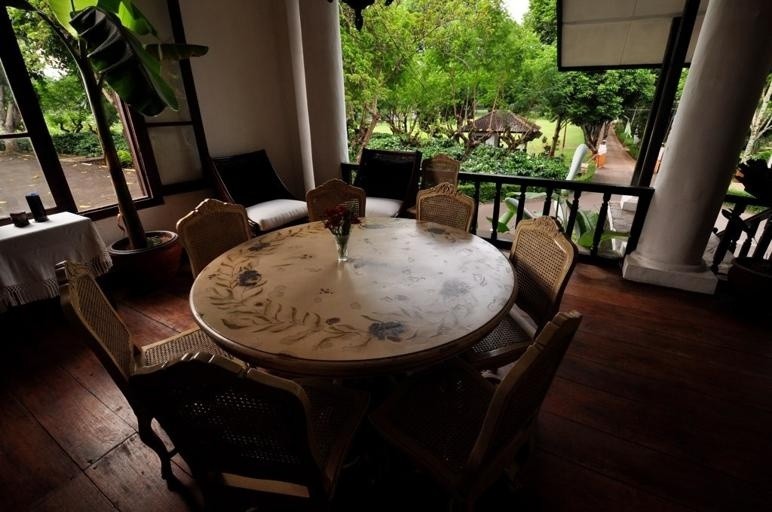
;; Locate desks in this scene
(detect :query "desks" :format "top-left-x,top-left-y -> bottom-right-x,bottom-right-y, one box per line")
0,210 -> 112,311
188,217 -> 518,370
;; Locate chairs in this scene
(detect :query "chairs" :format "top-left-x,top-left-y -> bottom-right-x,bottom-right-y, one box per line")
349,147 -> 460,219
305,176 -> 368,222
386,307 -> 582,506
414,182 -> 476,234
173,195 -> 248,274
471,214 -> 582,371
140,350 -> 342,510
210,149 -> 308,240
63,270 -> 224,480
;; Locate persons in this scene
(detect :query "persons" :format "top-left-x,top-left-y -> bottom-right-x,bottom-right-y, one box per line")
595,141 -> 609,169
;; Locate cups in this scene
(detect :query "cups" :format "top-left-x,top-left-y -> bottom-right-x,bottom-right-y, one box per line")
333,232 -> 350,262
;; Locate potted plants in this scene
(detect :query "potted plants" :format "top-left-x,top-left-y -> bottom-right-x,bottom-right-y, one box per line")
722,158 -> 771,308
0,0 -> 209,287
